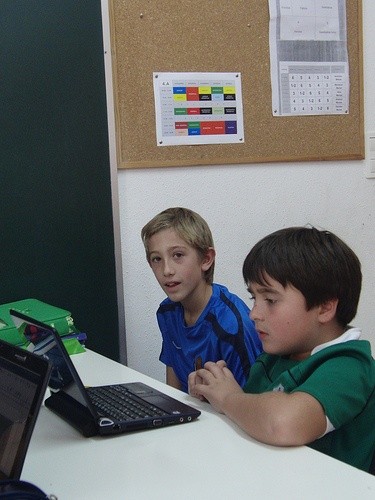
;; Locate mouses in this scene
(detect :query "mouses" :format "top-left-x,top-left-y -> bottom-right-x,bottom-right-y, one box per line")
0,480 -> 49,500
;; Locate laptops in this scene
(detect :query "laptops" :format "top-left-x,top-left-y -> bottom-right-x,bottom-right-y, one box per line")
9,309 -> 202,437
0,339 -> 53,482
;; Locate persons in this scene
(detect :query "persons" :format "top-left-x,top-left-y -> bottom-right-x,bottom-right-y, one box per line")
141,207 -> 265,394
188,226 -> 375,477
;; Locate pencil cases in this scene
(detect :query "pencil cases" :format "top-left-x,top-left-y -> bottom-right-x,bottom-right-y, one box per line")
0,298 -> 86,356
